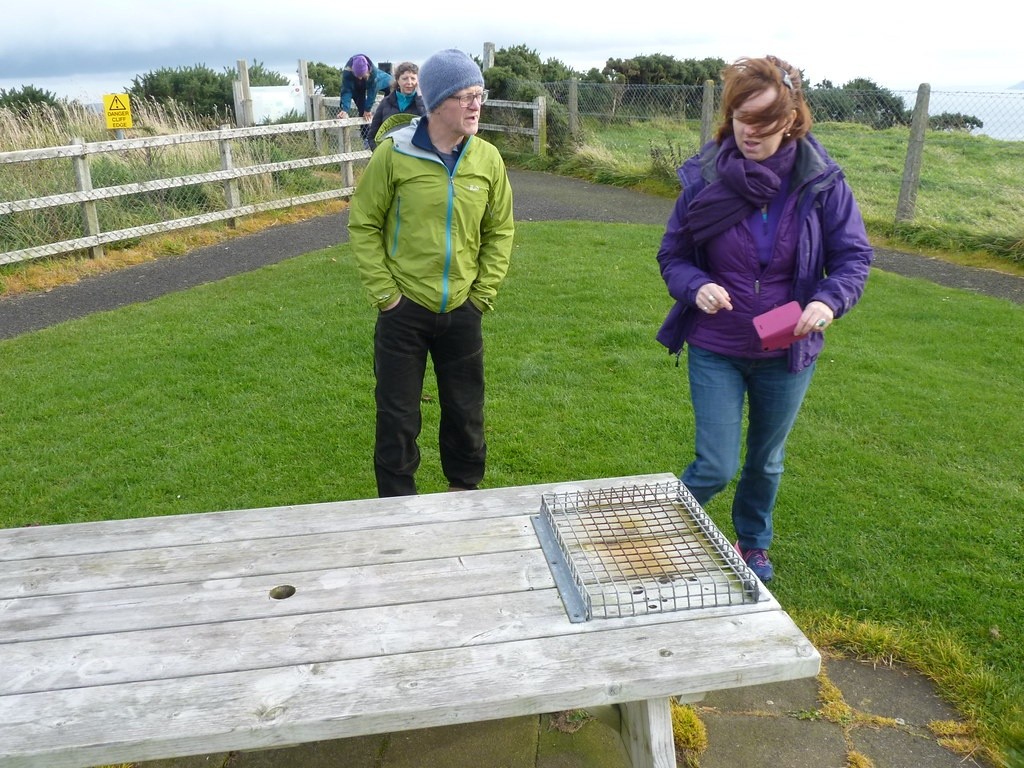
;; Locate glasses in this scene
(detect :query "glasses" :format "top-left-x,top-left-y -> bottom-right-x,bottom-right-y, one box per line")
774,65 -> 794,91
448,87 -> 489,107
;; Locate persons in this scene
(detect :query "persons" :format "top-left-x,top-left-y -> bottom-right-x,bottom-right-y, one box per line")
336,53 -> 396,150
656,54 -> 873,580
366,61 -> 429,152
345,48 -> 514,498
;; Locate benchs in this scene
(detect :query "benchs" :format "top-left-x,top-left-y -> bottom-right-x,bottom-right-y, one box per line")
0,470 -> 821,768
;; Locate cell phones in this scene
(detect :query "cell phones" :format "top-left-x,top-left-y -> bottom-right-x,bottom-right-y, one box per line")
753,299 -> 810,352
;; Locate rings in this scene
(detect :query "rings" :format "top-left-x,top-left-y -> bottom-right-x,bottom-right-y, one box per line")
703,306 -> 707,311
816,319 -> 827,328
708,295 -> 715,302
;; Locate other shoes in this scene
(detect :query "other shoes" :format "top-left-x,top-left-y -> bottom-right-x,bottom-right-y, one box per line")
363,138 -> 370,150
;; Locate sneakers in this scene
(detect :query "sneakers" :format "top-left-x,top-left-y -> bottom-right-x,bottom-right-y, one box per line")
735,540 -> 773,581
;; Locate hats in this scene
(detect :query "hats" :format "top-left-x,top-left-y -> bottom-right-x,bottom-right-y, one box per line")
352,56 -> 368,77
418,48 -> 485,112
